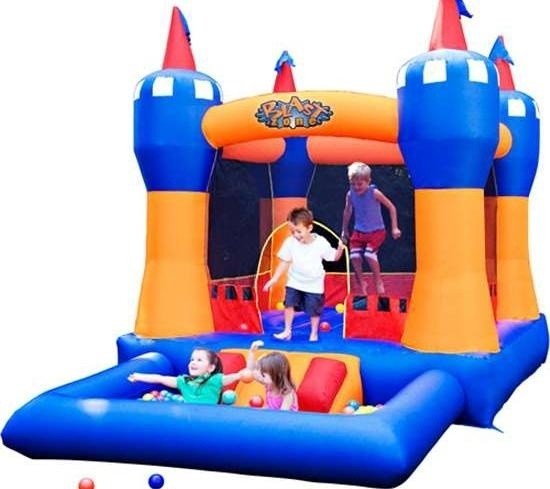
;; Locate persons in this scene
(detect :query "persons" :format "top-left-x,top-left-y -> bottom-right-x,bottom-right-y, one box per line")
335,161 -> 402,297
247,339 -> 300,412
261,205 -> 347,344
126,347 -> 251,407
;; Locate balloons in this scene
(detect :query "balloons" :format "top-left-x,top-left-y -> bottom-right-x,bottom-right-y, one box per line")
276,302 -> 284,311
148,473 -> 164,489
319,321 -> 331,331
343,400 -> 384,415
78,478 -> 95,489
222,389 -> 237,405
137,389 -> 183,402
248,393 -> 265,407
335,301 -> 346,314
